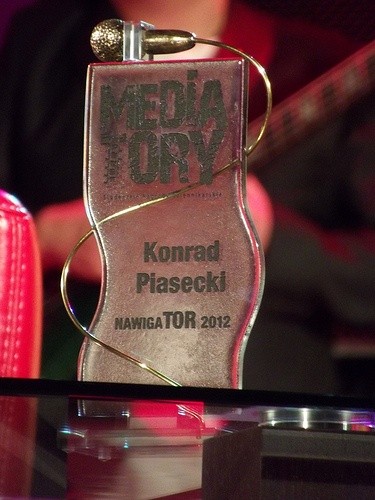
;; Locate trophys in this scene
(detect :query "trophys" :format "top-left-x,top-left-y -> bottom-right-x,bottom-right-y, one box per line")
60,18 -> 274,389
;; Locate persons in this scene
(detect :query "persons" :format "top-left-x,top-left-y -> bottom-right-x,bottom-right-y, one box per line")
0,0 -> 375,396
